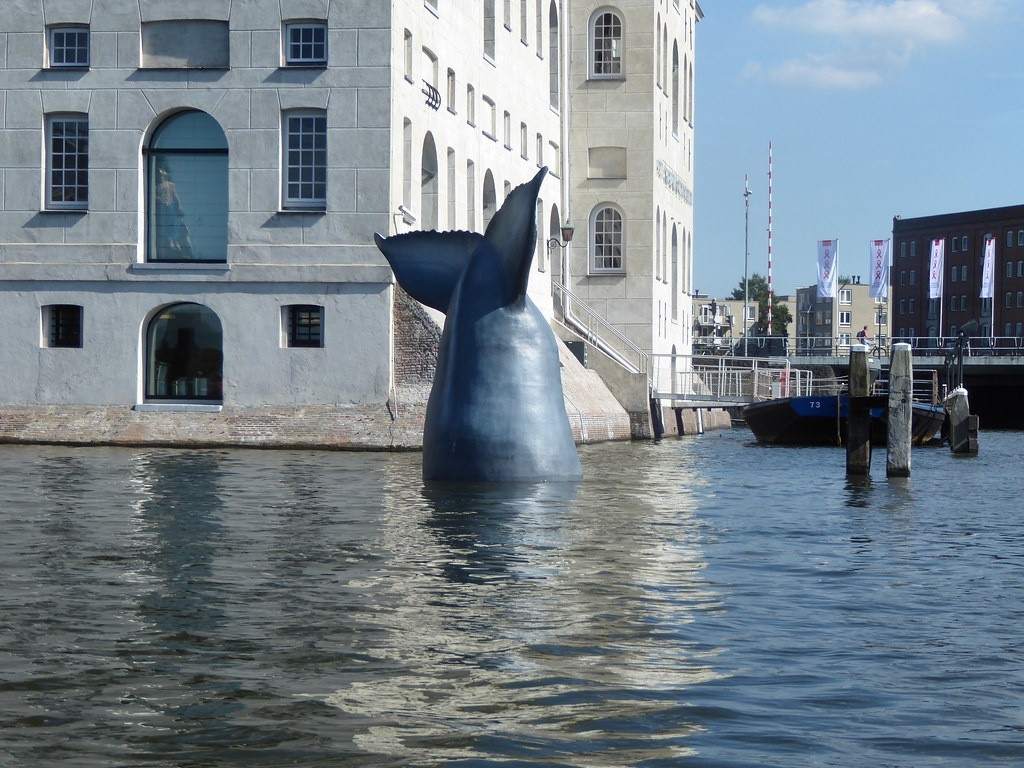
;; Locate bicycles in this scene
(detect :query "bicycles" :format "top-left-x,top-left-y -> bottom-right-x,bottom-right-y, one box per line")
869,339 -> 887,357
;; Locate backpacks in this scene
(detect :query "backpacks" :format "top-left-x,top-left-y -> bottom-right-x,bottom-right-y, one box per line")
856,331 -> 862,340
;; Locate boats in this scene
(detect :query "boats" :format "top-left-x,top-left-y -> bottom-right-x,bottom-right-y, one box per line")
743,359 -> 947,445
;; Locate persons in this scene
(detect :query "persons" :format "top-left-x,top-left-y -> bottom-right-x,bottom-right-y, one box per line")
859,325 -> 872,345
